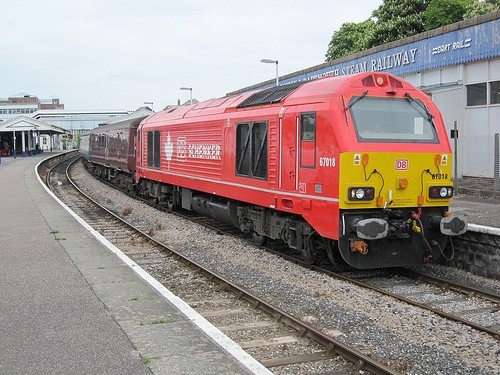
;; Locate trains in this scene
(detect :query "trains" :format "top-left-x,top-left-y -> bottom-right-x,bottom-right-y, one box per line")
76,70 -> 468,271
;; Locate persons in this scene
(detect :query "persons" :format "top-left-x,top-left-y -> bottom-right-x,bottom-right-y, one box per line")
35,142 -> 39,150
5,143 -> 11,156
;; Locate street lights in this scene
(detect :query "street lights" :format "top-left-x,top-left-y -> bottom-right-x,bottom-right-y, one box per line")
178,87 -> 193,103
261,58 -> 281,86
144,102 -> 153,111
